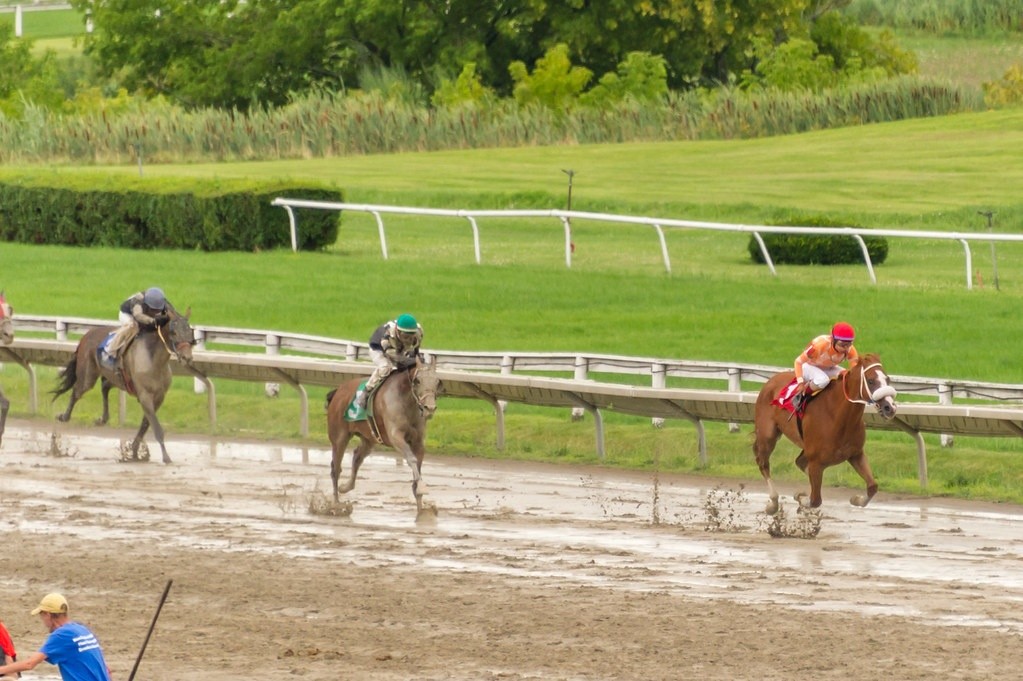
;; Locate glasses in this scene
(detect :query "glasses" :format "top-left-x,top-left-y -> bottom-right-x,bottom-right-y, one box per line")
399,331 -> 416,339
836,340 -> 853,348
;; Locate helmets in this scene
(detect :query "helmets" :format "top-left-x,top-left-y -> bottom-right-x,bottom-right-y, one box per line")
396,314 -> 418,334
144,287 -> 166,310
833,321 -> 855,341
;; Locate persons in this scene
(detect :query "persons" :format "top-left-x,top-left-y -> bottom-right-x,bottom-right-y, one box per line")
0,592 -> 109,681
792,322 -> 859,411
355,313 -> 426,410
0,622 -> 20,681
100,287 -> 175,367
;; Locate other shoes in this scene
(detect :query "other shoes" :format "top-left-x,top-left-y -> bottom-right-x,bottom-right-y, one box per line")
99,352 -> 113,370
357,389 -> 370,410
791,395 -> 802,412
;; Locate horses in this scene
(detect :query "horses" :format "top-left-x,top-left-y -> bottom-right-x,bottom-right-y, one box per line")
751,352 -> 898,516
325,355 -> 440,516
47,306 -> 195,466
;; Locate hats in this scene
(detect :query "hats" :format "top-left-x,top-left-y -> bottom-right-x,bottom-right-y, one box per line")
31,593 -> 68,616
